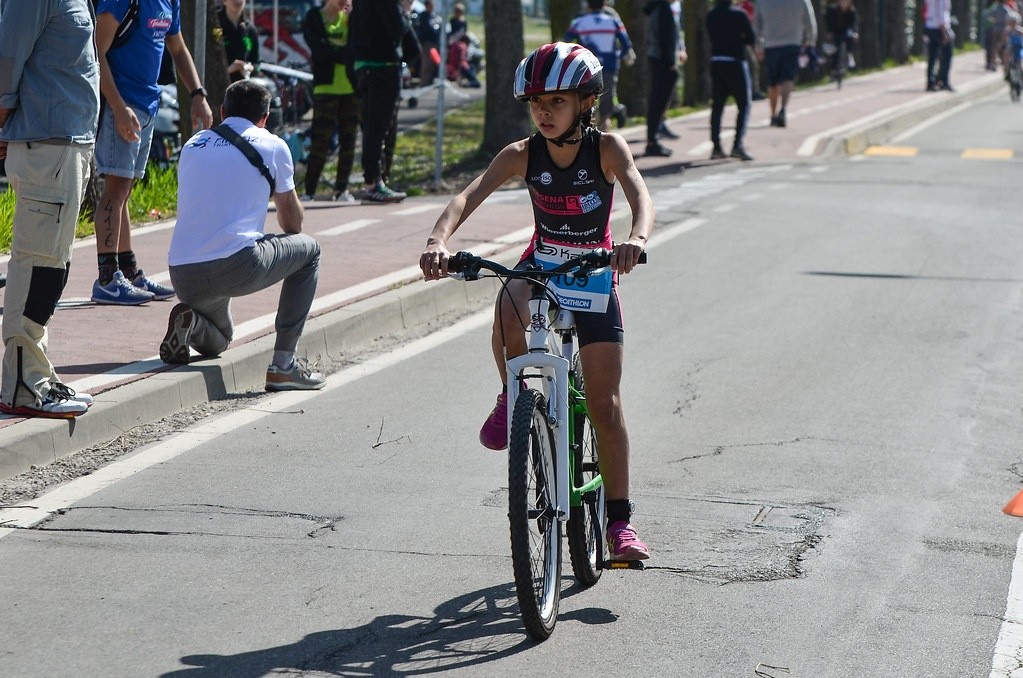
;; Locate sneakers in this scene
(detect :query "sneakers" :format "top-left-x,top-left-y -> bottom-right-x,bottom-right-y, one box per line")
360,179 -> 407,205
47,381 -> 94,407
479,381 -> 528,451
264,356 -> 328,390
0,389 -> 88,417
127,268 -> 175,299
160,302 -> 194,365
606,520 -> 650,561
90,270 -> 155,305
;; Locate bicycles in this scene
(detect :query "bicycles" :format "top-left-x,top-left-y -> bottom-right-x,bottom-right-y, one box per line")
826,28 -> 854,90
419,246 -> 646,640
999,44 -> 1022,102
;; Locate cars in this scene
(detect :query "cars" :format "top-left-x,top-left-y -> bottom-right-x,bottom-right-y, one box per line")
409,3 -> 482,74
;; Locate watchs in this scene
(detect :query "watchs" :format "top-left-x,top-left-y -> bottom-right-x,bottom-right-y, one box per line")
190,87 -> 207,97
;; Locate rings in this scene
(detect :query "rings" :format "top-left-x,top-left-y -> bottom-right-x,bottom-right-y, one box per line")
127,129 -> 130,132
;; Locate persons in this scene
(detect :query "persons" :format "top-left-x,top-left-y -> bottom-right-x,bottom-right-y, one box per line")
923,0 -> 955,93
213,0 -> 260,82
301,0 -> 423,204
414,0 -> 480,88
160,80 -> 327,390
982,0 -> 1023,83
420,39 -> 654,563
706,0 -> 818,161
565,1 -> 636,130
645,0 -> 687,157
825,0 -> 860,66
87,0 -> 212,306
1,0 -> 93,415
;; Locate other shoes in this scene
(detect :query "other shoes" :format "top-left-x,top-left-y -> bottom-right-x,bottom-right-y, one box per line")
771,111 -> 785,128
926,76 -> 952,91
752,90 -> 767,101
845,51 -> 857,68
332,190 -> 356,202
656,124 -> 679,140
730,144 -> 752,161
301,194 -> 316,202
711,145 -> 730,160
613,107 -> 627,128
644,144 -> 673,157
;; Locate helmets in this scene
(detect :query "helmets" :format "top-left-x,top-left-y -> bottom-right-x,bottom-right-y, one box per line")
512,41 -> 605,101
